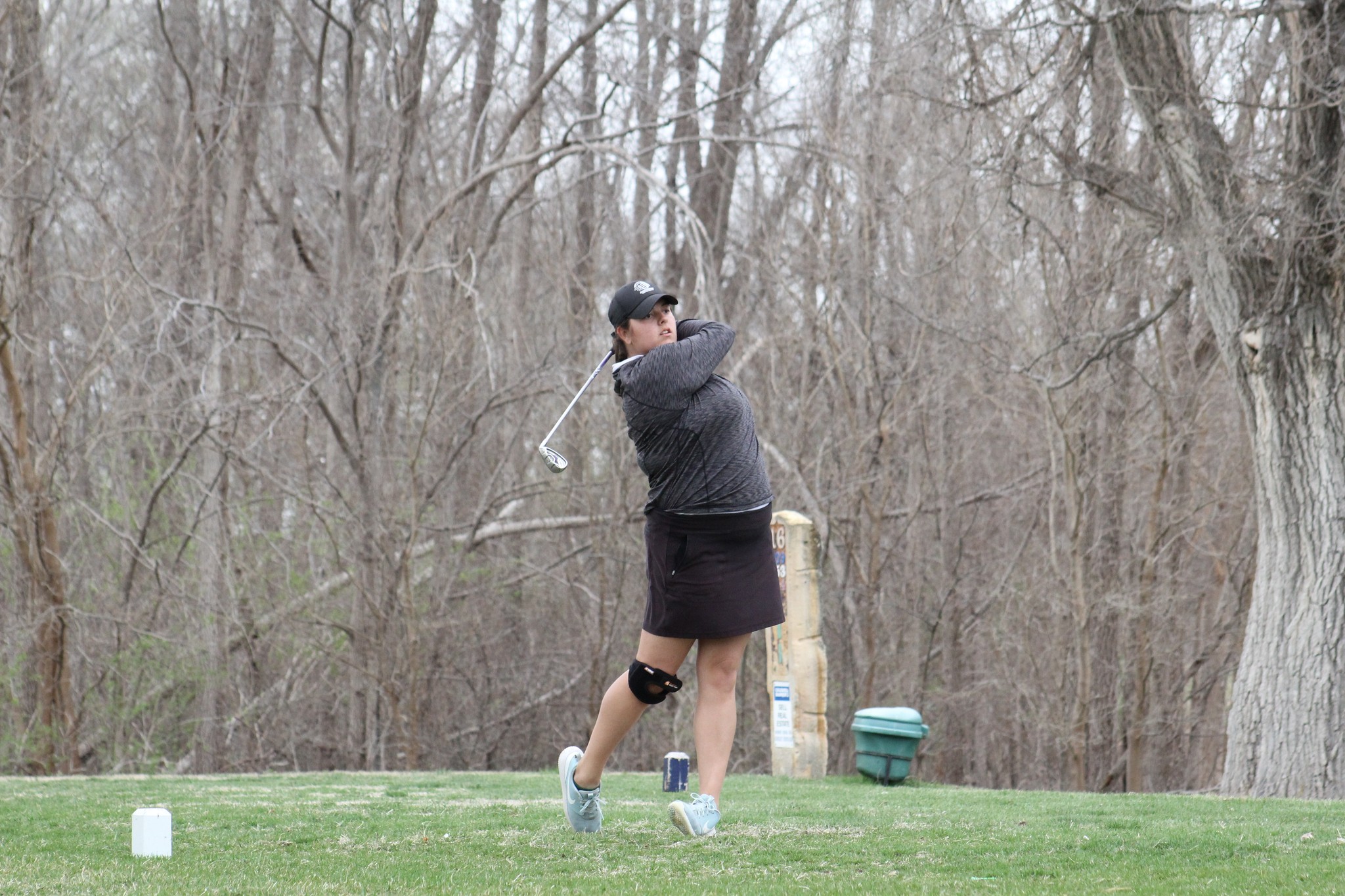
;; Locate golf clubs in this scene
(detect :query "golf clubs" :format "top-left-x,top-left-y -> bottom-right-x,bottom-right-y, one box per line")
537,346 -> 615,474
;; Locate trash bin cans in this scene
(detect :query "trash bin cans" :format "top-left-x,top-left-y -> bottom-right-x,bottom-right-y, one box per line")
850,706 -> 928,780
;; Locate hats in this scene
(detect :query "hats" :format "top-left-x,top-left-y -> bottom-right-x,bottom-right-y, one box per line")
608,280 -> 678,329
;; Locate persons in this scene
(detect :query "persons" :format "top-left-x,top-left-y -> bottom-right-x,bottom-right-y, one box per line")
558,281 -> 785,838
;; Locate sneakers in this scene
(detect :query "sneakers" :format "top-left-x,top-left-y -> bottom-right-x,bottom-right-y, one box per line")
667,793 -> 721,837
558,746 -> 606,833
